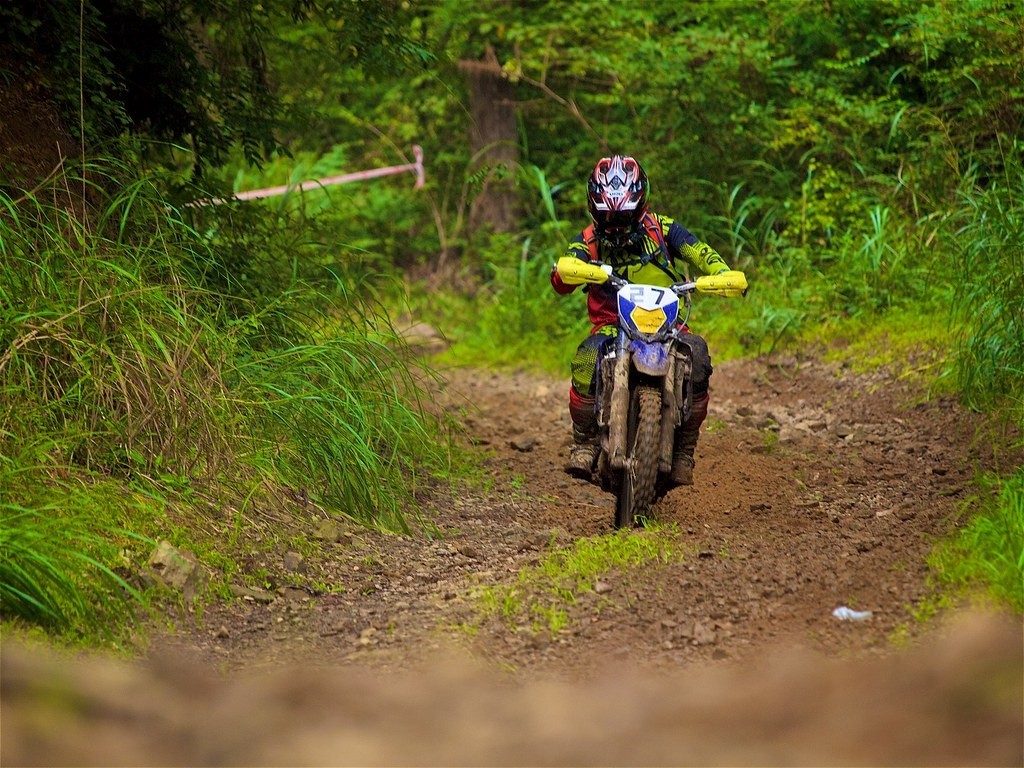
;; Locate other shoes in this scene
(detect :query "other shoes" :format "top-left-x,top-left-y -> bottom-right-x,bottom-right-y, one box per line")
671,448 -> 696,482
564,438 -> 600,475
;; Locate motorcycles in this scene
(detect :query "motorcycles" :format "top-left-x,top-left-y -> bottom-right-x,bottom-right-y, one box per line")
555,255 -> 749,536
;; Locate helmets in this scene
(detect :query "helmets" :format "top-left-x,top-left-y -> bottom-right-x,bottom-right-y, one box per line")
587,154 -> 649,240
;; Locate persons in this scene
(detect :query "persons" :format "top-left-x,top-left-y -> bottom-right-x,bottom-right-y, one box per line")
551,155 -> 733,487
